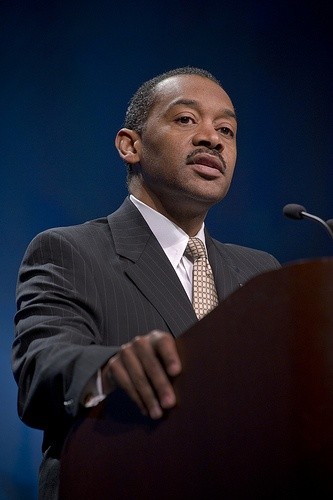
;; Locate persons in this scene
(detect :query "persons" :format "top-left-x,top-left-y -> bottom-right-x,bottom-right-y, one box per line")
11,67 -> 285,500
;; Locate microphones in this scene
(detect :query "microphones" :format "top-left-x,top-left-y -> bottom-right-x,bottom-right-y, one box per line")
284,204 -> 333,237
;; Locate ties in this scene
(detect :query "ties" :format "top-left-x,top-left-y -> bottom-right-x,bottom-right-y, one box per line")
183,238 -> 219,321
